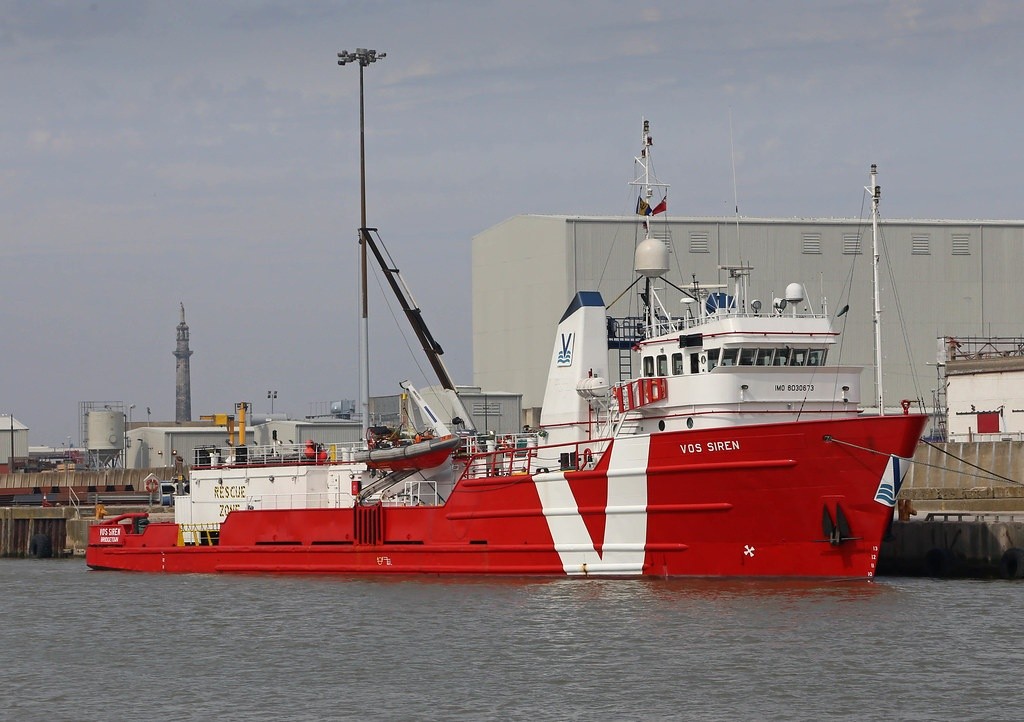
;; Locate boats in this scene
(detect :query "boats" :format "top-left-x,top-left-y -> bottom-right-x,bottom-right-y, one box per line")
354,378 -> 461,470
84,46 -> 932,580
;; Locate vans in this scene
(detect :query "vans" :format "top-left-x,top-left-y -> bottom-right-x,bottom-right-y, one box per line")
156,478 -> 189,507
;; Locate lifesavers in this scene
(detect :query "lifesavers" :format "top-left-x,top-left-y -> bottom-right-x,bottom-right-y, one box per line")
497,433 -> 516,448
642,380 -> 666,401
146,479 -> 159,491
